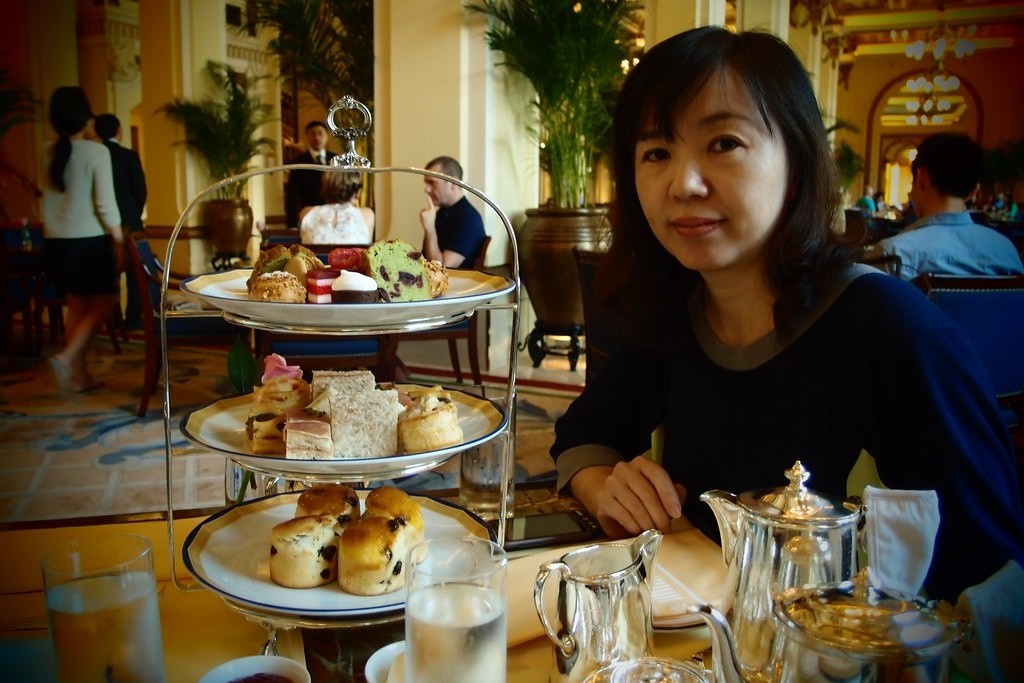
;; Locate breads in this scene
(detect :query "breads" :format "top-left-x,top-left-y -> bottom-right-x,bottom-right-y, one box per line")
245,237 -> 450,304
270,483 -> 427,596
245,354 -> 463,462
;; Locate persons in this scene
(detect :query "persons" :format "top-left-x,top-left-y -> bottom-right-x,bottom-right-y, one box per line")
38,85 -> 126,390
549,26 -> 1024,683
95,114 -> 147,230
968,192 -> 1018,216
868,130 -> 1024,428
285,121 -> 340,232
300,171 -> 410,383
420,156 -> 486,270
857,185 -> 917,243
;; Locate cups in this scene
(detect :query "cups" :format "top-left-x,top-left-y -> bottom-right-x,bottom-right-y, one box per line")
40,533 -> 165,683
460,385 -> 516,520
700,461 -> 867,683
405,536 -> 508,683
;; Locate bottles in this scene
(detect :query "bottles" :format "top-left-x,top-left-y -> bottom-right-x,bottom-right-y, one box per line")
21,217 -> 32,251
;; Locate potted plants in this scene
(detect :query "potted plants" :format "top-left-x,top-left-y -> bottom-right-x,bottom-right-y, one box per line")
464,3 -> 647,332
152,61 -> 282,257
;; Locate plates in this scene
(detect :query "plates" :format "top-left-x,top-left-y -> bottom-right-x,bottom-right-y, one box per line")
179,391 -> 509,473
182,490 -> 499,617
179,267 -> 515,325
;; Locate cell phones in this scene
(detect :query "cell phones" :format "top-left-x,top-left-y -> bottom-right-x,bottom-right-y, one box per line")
487,509 -> 601,553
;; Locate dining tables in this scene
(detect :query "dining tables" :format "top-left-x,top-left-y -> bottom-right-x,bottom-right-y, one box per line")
0,470 -> 728,683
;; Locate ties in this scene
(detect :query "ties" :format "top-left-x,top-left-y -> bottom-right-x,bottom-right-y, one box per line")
317,154 -> 323,165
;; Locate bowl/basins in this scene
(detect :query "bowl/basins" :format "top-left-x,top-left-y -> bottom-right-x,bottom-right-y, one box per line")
365,640 -> 405,683
198,655 -> 311,683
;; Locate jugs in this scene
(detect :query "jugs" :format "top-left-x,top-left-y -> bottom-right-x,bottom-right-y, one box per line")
686,567 -> 975,683
534,529 -> 664,683
583,655 -> 709,683
